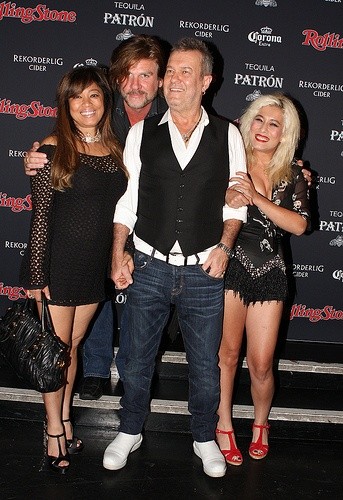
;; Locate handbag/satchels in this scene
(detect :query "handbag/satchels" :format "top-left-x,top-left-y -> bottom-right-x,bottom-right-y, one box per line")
0,292 -> 72,393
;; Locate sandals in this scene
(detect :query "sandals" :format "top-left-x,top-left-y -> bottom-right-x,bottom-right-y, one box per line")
215,428 -> 243,466
248,423 -> 270,459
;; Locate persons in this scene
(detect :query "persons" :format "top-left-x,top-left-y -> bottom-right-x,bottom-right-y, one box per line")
24,36 -> 312,477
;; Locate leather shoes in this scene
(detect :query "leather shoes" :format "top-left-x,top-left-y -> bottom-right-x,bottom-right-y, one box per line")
79,377 -> 103,400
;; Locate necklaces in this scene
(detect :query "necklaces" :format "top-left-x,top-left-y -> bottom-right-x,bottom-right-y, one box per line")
77,128 -> 102,144
255,153 -> 268,176
182,110 -> 203,143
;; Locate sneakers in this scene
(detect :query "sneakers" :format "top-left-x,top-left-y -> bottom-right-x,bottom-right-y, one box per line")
193,440 -> 227,477
103,433 -> 142,470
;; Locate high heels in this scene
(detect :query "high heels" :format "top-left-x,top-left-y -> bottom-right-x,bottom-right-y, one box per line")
45,425 -> 70,469
45,415 -> 82,449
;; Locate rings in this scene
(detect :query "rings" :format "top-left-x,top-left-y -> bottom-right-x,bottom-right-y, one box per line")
29,294 -> 33,298
25,157 -> 28,163
221,271 -> 226,274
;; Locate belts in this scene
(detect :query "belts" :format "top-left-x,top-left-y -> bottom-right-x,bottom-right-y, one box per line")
133,231 -> 218,267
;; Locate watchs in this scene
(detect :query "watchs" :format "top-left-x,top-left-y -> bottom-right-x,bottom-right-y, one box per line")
217,242 -> 235,260
